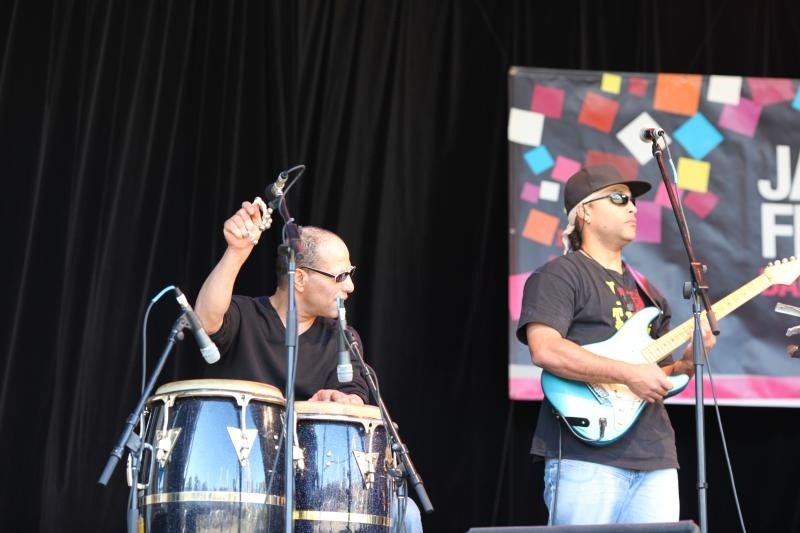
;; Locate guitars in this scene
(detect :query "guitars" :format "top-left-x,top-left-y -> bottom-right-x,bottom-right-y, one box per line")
541,256 -> 800,448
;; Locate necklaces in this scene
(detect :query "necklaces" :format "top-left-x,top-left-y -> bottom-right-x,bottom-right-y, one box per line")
581,245 -> 635,313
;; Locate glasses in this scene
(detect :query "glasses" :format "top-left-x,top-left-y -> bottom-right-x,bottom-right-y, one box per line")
581,193 -> 636,207
299,265 -> 356,283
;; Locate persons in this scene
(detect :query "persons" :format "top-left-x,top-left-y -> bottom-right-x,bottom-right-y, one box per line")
191,201 -> 423,533
515,164 -> 716,526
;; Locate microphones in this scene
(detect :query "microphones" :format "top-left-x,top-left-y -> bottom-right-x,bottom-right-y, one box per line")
336,294 -> 353,382
175,287 -> 220,364
639,127 -> 665,143
265,171 -> 288,200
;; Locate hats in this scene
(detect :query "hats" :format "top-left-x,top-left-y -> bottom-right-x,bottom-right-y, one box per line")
564,163 -> 652,216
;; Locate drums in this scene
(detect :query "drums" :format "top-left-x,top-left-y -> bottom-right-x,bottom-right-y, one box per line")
136,379 -> 286,533
293,400 -> 392,533
